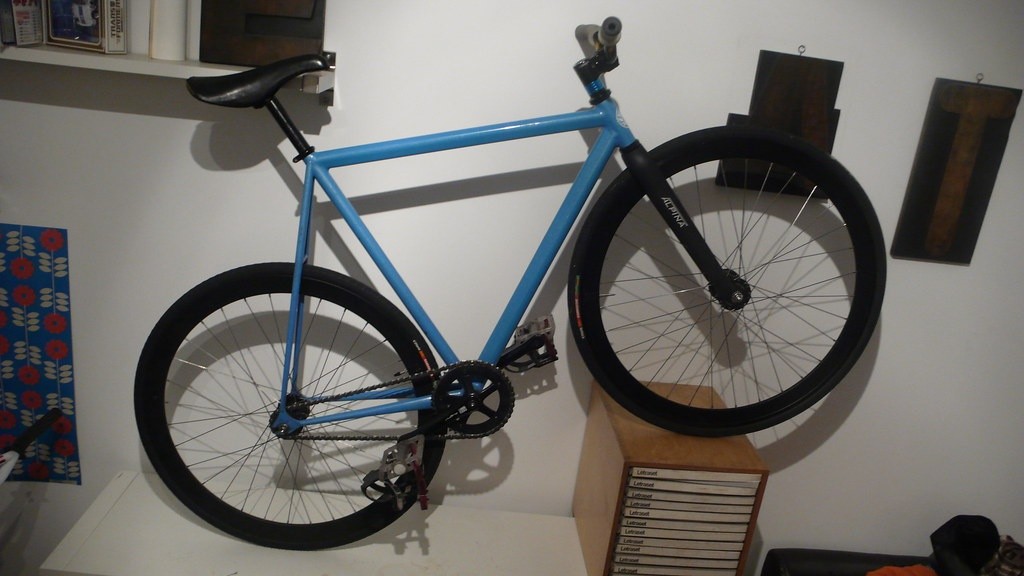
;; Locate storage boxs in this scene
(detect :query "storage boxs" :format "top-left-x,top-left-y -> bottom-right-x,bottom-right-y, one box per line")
41,0 -> 127,55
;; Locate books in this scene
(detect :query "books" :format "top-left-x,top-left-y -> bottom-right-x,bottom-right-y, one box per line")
0,0 -> 128,55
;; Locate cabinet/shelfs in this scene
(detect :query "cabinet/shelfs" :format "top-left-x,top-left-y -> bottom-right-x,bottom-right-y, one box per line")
36,470 -> 588,576
573,380 -> 770,576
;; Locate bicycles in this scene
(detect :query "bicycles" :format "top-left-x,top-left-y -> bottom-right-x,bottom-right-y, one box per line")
129,15 -> 891,551
